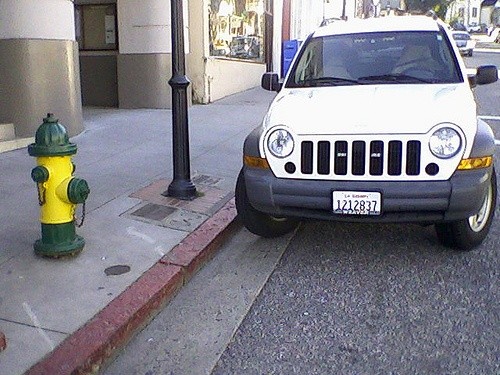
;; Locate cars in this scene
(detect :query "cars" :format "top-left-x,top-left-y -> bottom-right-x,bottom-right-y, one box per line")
449,30 -> 475,55
235,14 -> 497,251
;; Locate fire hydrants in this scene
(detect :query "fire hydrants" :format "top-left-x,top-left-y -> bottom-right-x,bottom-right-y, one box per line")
27,111 -> 89,257
